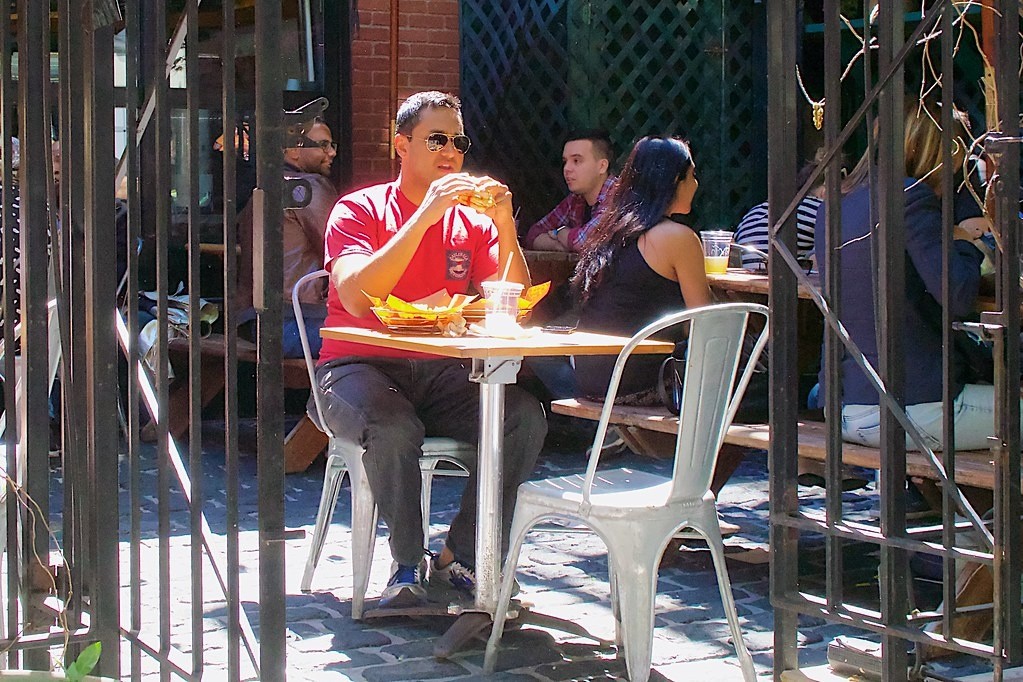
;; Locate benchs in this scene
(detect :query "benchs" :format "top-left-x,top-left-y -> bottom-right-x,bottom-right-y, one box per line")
550,398 -> 994,661
140,333 -> 330,474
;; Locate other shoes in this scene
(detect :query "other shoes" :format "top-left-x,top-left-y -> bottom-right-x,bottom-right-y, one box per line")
869,484 -> 944,521
922,561 -> 995,660
586,428 -> 626,461
718,513 -> 741,540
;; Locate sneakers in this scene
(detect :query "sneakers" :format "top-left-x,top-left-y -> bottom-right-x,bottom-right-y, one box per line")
377,558 -> 429,608
431,551 -> 521,599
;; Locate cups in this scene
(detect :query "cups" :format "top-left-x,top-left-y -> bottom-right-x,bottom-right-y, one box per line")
481,281 -> 524,338
514,218 -> 520,234
699,229 -> 734,275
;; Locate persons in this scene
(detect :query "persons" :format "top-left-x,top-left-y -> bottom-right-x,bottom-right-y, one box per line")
235,120 -> 339,357
813,95 -> 1023,577
314,88 -> 549,609
566,134 -> 747,534
522,124 -> 629,460
731,145 -> 848,269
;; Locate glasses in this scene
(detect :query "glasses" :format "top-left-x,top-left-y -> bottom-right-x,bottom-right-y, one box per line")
316,140 -> 338,153
404,133 -> 471,156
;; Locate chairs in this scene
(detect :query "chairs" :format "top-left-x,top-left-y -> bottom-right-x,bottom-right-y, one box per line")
292,270 -> 478,620
482,302 -> 769,682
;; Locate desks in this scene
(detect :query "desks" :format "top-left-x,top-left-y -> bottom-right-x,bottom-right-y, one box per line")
140,244 -> 582,473
661,267 -> 1023,661
320,325 -> 676,672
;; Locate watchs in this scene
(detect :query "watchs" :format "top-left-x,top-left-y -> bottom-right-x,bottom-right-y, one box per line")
554,224 -> 568,239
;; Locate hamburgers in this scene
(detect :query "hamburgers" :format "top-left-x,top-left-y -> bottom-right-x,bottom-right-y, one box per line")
459,189 -> 496,213
